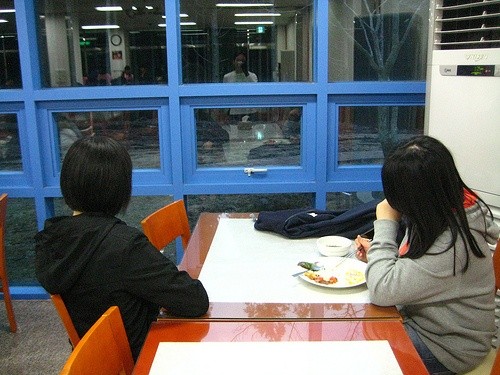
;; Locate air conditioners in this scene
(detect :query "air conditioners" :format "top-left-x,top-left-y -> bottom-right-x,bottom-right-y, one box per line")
353,12 -> 422,130
426,0 -> 500,351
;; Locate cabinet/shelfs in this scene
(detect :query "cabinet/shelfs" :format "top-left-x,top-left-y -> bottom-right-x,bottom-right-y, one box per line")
281,50 -> 296,83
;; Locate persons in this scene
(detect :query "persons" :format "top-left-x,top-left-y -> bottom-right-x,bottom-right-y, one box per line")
78,64 -> 165,86
355,135 -> 500,375
33,135 -> 210,363
223,55 -> 259,123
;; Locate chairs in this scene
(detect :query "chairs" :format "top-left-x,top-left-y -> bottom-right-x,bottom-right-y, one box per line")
60,306 -> 135,375
141,200 -> 192,252
462,237 -> 500,375
0,194 -> 17,333
50,292 -> 80,350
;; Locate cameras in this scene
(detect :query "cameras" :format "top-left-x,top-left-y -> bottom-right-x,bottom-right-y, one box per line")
237,61 -> 243,67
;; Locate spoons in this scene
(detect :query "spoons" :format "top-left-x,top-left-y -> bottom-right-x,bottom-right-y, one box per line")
292,266 -> 320,277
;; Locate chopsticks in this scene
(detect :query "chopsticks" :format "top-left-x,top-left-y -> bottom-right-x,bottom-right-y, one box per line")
336,239 -> 372,268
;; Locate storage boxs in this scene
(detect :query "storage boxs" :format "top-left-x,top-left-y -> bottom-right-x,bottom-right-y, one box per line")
237,121 -> 252,130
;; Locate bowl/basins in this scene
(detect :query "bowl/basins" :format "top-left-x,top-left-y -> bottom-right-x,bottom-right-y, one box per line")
317,236 -> 353,257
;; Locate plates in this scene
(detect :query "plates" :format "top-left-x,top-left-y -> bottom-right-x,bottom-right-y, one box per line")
295,256 -> 367,288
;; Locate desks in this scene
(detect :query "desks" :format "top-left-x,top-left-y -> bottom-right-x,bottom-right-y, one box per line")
127,209 -> 428,375
211,124 -> 290,167
